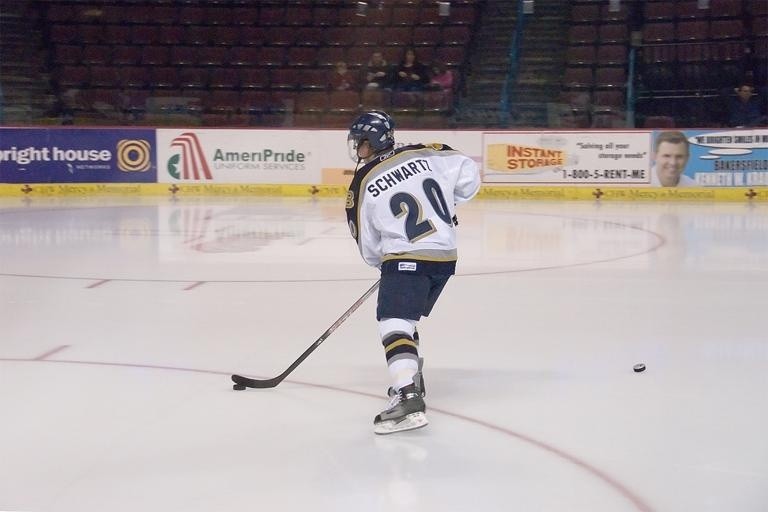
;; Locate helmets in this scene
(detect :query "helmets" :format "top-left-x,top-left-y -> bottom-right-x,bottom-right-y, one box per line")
346,110 -> 395,165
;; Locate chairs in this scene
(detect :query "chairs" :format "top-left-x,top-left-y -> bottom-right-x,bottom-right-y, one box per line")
0,0 -> 768,131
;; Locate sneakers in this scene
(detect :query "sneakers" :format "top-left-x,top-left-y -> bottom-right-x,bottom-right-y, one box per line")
373,387 -> 426,422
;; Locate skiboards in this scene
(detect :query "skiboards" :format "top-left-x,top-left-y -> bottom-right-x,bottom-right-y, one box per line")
231,278 -> 380,389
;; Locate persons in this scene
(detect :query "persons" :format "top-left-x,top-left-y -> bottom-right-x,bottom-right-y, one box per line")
328,47 -> 453,92
718,81 -> 768,126
650,132 -> 694,187
345,109 -> 482,427
